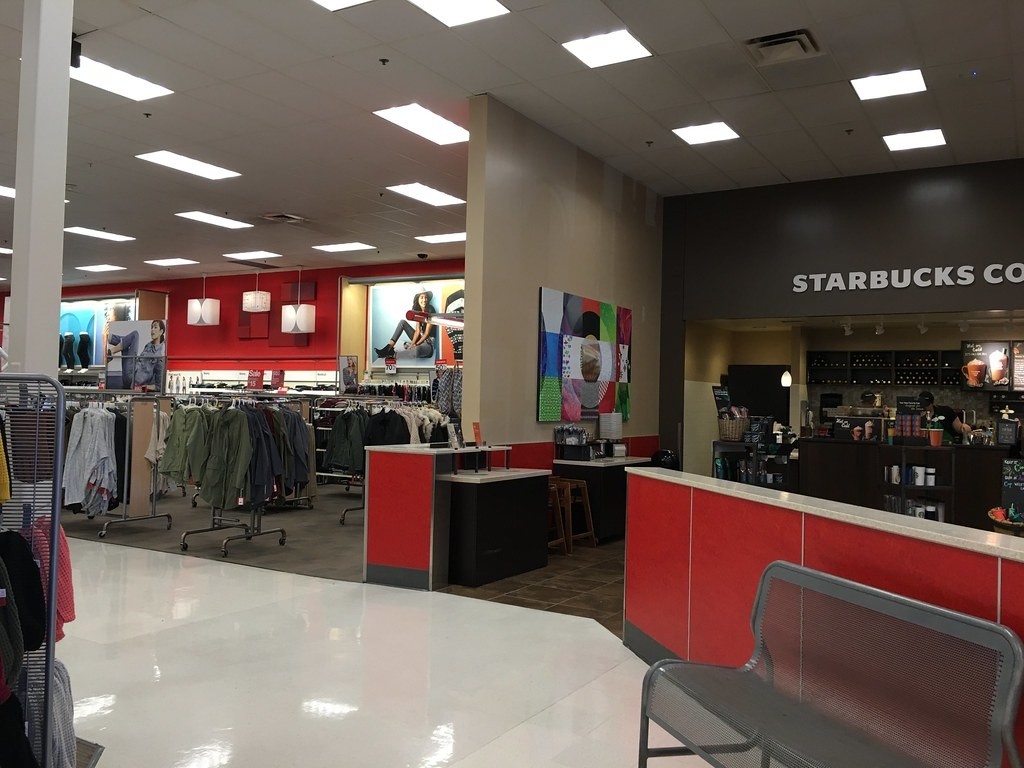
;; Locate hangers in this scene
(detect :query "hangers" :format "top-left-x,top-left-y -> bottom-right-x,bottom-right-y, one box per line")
64,380 -> 435,416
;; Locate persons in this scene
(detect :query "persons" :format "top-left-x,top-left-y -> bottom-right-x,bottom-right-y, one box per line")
103,303 -> 131,365
77,332 -> 90,373
63,333 -> 75,374
375,287 -> 437,358
343,356 -> 358,394
168,376 -> 199,394
917,392 -> 971,445
58,333 -> 64,372
108,320 -> 166,391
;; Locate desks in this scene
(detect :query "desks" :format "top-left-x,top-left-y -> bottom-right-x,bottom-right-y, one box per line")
711,441 -> 790,485
448,445 -> 552,588
553,457 -> 651,546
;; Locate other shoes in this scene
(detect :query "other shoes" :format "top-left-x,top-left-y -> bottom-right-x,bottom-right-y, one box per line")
107,349 -> 113,363
404,342 -> 410,349
374,344 -> 394,360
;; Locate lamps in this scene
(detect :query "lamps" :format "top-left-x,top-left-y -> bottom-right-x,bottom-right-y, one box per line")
281,265 -> 315,333
188,273 -> 220,326
1003,318 -> 1017,332
242,271 -> 270,312
959,318 -> 969,332
874,321 -> 885,335
844,323 -> 854,336
917,320 -> 928,334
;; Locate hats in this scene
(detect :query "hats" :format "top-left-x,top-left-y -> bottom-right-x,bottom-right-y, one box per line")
413,287 -> 433,303
917,391 -> 934,408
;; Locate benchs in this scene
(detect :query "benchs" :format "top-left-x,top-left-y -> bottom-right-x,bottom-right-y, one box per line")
638,560 -> 1024,768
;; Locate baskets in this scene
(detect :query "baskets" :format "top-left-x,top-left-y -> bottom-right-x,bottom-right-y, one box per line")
717,416 -> 750,441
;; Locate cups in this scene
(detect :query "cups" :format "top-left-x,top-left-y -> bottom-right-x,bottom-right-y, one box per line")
850,426 -> 863,440
912,468 -> 936,486
865,421 -> 874,439
885,466 -> 899,485
990,356 -> 1007,381
921,428 -> 943,446
962,364 -> 985,387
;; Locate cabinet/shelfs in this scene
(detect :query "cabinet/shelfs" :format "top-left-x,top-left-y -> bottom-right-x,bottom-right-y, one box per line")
806,340 -> 1024,415
878,444 -> 956,521
